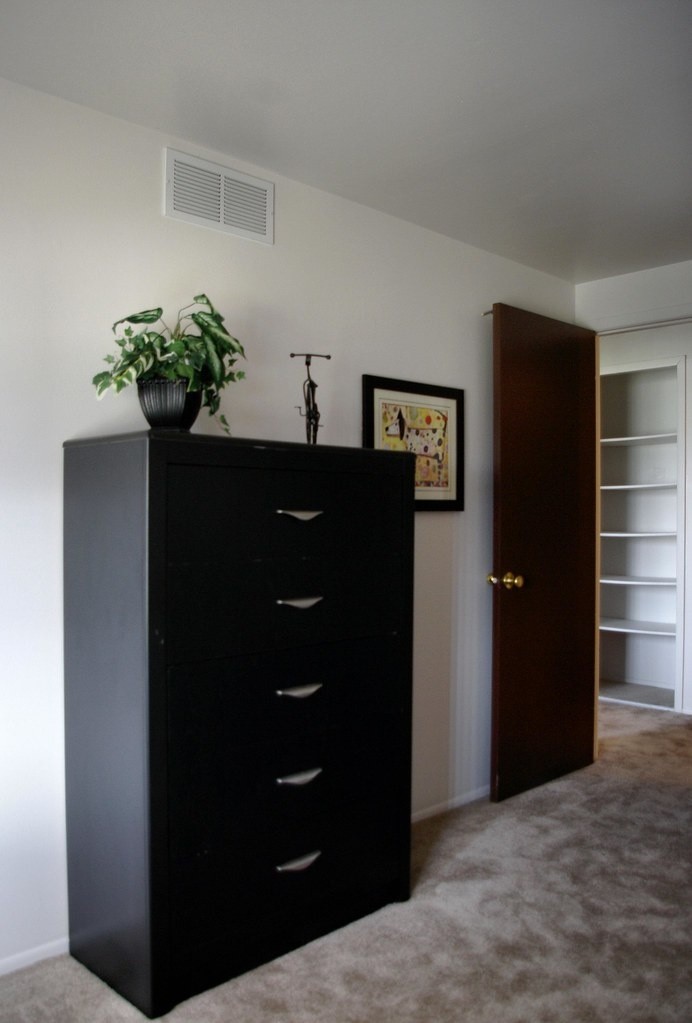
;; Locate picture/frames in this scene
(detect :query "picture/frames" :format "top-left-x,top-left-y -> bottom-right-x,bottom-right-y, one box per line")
360,373 -> 466,514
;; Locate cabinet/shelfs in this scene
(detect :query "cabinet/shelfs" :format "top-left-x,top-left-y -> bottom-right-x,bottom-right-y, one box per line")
594,358 -> 688,714
60,429 -> 414,1019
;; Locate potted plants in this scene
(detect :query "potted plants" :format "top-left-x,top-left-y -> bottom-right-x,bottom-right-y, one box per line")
90,291 -> 247,433
